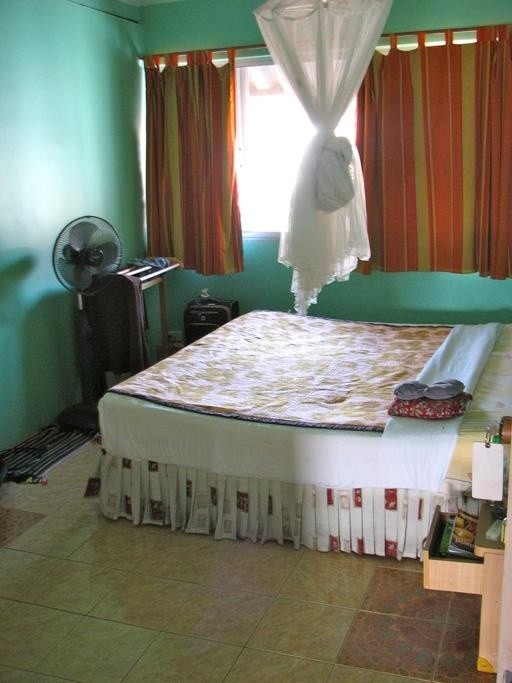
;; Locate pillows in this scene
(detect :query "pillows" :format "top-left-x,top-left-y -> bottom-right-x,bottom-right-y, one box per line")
388,393 -> 472,420
394,379 -> 463,399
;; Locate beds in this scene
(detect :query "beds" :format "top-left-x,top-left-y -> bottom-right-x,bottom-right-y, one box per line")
84,309 -> 512,563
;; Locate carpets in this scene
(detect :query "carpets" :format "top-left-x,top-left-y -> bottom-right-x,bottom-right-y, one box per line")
0,422 -> 98,485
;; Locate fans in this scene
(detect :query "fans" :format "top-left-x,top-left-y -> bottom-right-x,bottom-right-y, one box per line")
51,214 -> 123,432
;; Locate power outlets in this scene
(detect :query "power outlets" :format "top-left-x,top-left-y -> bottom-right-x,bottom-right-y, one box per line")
167,331 -> 184,342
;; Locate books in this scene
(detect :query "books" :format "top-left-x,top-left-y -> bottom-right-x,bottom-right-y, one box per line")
439,509 -> 482,560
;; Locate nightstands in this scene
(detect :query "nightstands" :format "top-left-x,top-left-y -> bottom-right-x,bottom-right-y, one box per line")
423,491 -> 504,675
